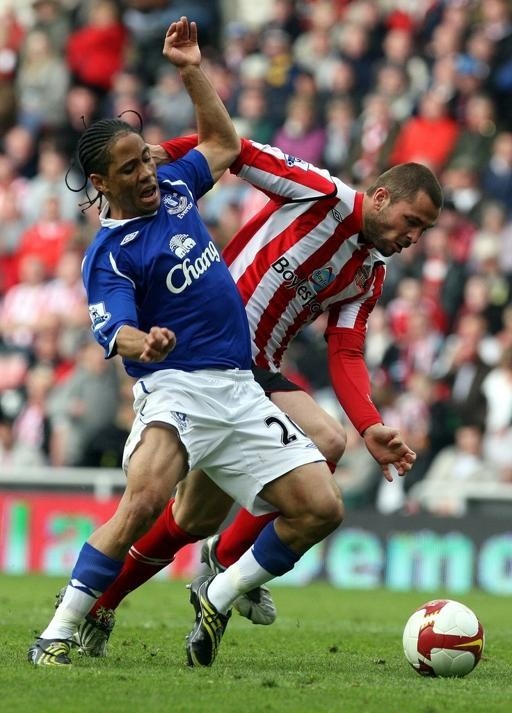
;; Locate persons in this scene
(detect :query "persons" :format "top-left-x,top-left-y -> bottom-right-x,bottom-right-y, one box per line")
54,132 -> 443,658
1,1 -> 512,518
27,14 -> 345,668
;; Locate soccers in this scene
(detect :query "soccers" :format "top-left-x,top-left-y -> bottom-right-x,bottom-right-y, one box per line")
403,599 -> 485,678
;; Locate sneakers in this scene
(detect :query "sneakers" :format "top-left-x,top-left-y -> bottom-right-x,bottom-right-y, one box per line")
54,583 -> 115,659
186,576 -> 231,665
28,638 -> 86,670
202,532 -> 277,626
